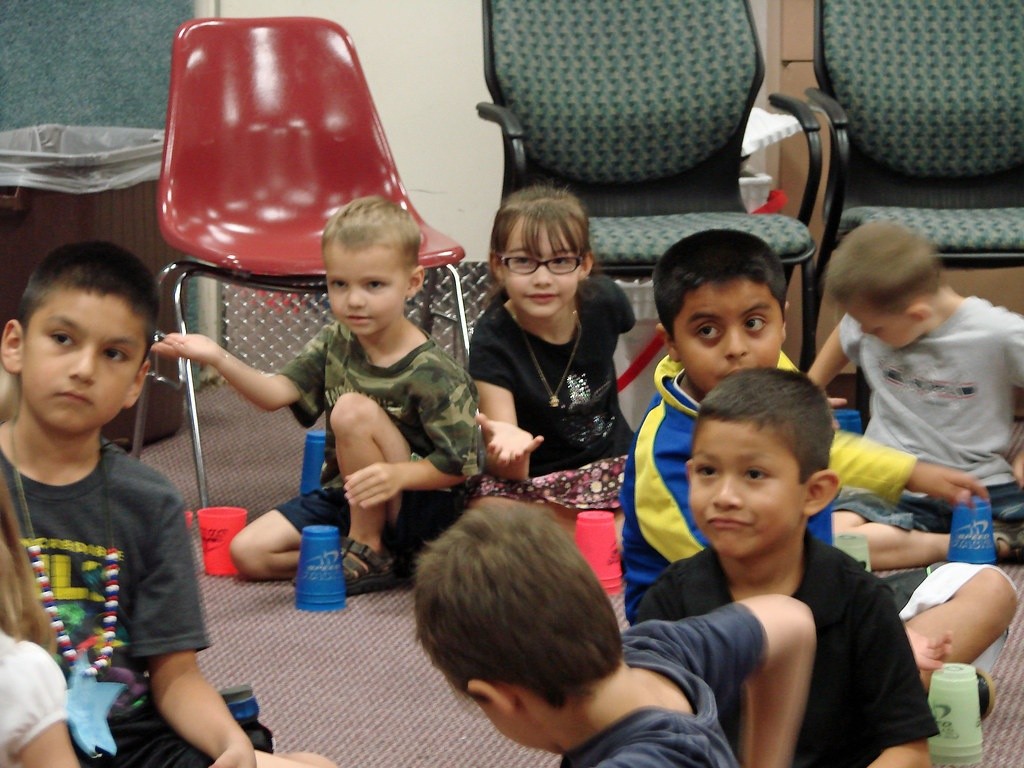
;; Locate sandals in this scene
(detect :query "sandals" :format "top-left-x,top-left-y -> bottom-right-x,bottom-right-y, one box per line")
291,537 -> 405,595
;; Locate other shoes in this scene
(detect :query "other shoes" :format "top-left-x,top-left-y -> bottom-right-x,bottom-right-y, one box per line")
219,684 -> 259,723
976,667 -> 996,720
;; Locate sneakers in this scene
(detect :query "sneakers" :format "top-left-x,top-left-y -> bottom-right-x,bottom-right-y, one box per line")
993,521 -> 1024,565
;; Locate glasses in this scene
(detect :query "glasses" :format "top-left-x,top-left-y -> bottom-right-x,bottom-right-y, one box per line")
496,250 -> 588,275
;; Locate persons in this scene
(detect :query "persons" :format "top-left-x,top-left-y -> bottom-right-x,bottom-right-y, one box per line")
808,221 -> 1024,564
625,367 -> 940,767
0,465 -> 80,767
0,238 -> 336,768
467,188 -> 640,552
617,227 -> 1019,716
151,196 -> 483,597
414,502 -> 818,768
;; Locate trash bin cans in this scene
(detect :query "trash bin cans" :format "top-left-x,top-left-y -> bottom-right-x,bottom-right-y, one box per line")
0,123 -> 189,447
607,103 -> 801,433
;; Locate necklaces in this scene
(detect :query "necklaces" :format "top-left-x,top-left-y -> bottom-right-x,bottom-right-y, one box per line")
510,303 -> 581,408
11,422 -> 121,677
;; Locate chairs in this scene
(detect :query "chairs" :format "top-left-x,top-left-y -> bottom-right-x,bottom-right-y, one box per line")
476,0 -> 822,373
800,0 -> 1024,434
132,16 -> 469,510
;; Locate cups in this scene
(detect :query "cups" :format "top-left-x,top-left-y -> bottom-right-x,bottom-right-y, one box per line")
295,525 -> 347,612
834,531 -> 872,575
573,510 -> 623,595
945,496 -> 997,565
300,429 -> 327,496
195,506 -> 250,576
927,660 -> 985,765
